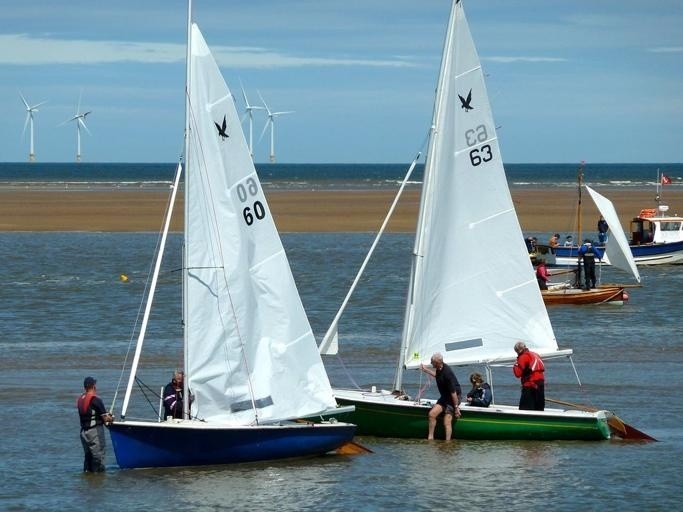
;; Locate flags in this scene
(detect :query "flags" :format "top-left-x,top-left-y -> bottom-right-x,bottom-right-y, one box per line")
661,174 -> 672,187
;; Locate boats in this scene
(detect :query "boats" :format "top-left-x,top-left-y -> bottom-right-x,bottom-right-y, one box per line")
529,216 -> 683,267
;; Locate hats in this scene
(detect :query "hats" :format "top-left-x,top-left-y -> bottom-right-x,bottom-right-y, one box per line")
84,376 -> 97,387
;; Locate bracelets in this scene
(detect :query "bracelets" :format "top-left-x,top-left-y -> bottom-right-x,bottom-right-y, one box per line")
453,403 -> 458,409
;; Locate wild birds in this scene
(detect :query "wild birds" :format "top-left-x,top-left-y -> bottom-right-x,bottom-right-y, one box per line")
458,88 -> 473,112
214,116 -> 229,141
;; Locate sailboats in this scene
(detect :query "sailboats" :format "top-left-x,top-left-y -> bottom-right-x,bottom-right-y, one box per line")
334,0 -> 612,439
104,0 -> 357,471
540,167 -> 645,304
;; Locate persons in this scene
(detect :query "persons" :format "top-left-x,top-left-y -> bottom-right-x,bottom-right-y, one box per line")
530,237 -> 536,253
464,372 -> 492,407
563,235 -> 573,249
512,341 -> 545,411
576,238 -> 603,291
535,257 -> 552,289
597,214 -> 608,244
419,353 -> 462,440
525,235 -> 533,253
162,370 -> 195,421
76,376 -> 114,473
548,233 -> 560,249
